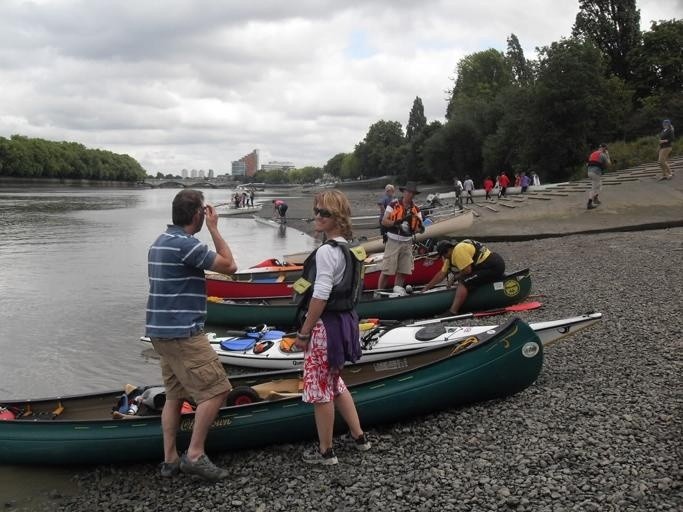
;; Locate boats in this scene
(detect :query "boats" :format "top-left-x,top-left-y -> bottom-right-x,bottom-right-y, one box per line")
434,181 -> 571,198
234,183 -> 265,193
212,203 -> 263,216
199,267 -> 533,329
0,316 -> 542,471
134,312 -> 605,368
204,206 -> 476,298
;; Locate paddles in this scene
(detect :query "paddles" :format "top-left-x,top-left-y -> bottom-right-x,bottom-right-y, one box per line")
403,301 -> 543,326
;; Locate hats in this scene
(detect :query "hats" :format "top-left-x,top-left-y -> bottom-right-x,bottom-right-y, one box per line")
436,240 -> 449,255
399,180 -> 420,194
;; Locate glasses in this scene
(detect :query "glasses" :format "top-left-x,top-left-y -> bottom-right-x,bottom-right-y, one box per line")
312,207 -> 331,217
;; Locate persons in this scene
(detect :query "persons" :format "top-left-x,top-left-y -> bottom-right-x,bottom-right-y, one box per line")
421,239 -> 503,317
378,185 -> 397,242
426,170 -> 542,211
292,187 -> 371,468
142,188 -> 239,481
657,120 -> 674,181
375,182 -> 424,301
272,199 -> 289,222
231,187 -> 255,209
585,142 -> 611,209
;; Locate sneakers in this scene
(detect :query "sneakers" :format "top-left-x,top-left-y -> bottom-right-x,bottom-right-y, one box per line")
160,457 -> 180,477
434,310 -> 454,319
594,199 -> 601,203
352,433 -> 371,451
588,203 -> 597,209
179,449 -> 229,482
301,445 -> 338,465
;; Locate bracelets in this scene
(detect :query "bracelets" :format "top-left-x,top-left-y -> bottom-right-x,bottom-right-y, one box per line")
295,330 -> 311,340
